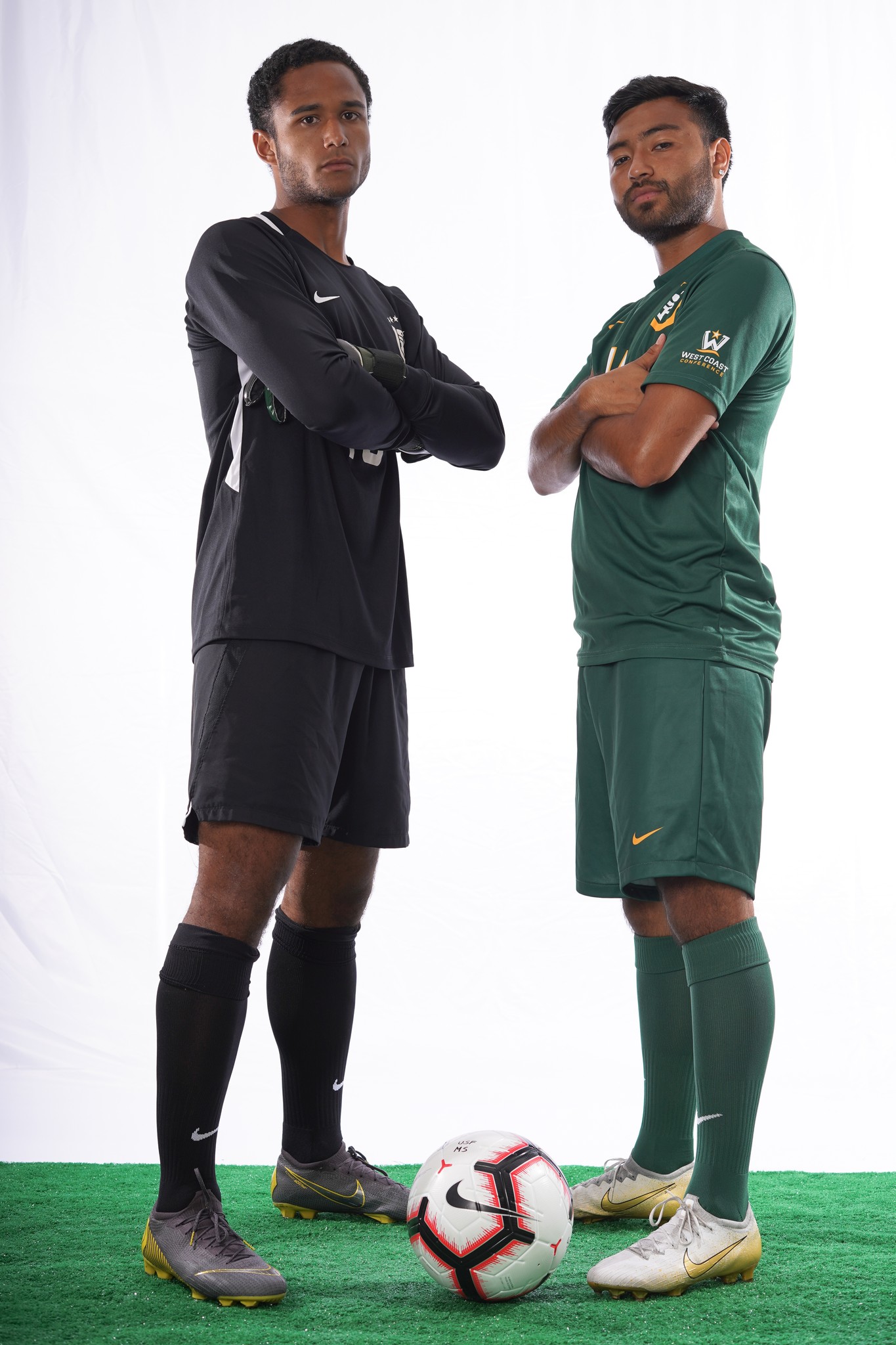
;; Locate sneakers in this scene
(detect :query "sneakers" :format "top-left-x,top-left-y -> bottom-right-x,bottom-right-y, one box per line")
270,1142 -> 410,1224
570,1154 -> 696,1220
587,1194 -> 762,1301
139,1169 -> 288,1306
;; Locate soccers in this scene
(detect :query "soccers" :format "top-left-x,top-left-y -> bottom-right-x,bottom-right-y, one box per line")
406,1129 -> 575,1303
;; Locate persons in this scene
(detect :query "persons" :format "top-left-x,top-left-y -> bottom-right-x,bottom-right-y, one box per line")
139,35 -> 504,1310
530,78 -> 795,1303
